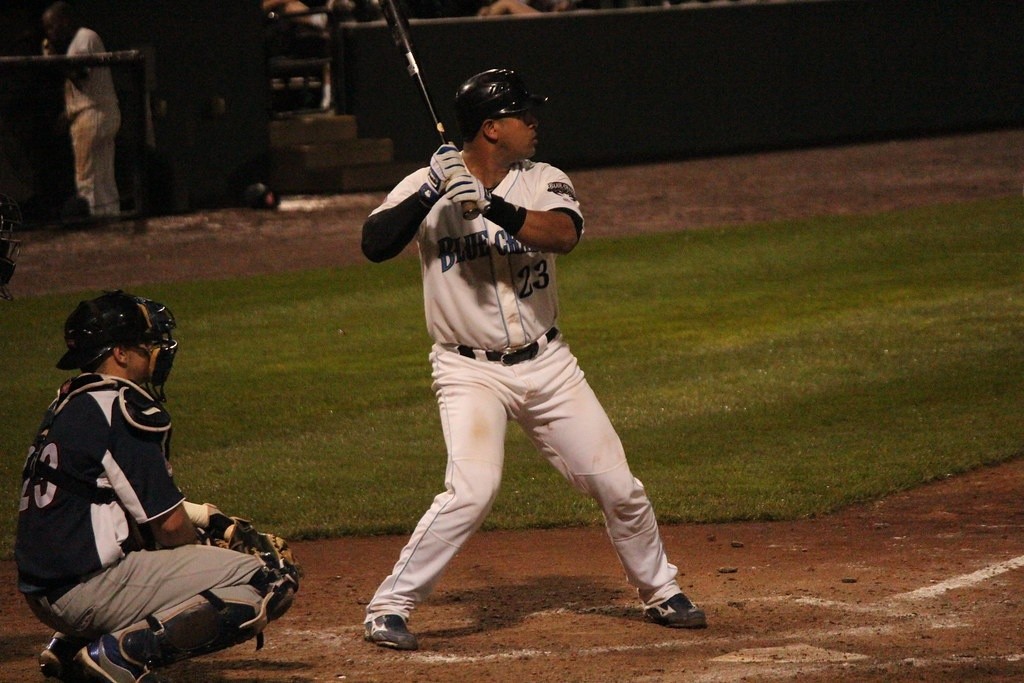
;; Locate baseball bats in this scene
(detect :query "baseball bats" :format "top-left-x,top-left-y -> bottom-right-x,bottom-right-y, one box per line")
380,0 -> 483,224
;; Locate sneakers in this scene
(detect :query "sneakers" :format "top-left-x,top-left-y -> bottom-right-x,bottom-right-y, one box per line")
644,594 -> 706,628
362,614 -> 417,652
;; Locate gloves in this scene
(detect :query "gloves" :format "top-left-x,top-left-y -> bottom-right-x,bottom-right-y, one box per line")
445,170 -> 528,237
417,143 -> 466,208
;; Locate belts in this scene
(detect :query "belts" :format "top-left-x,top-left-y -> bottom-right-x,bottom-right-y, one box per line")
458,326 -> 558,366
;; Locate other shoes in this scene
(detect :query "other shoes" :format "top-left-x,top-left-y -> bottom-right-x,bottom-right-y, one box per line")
38,629 -> 90,683
73,629 -> 156,683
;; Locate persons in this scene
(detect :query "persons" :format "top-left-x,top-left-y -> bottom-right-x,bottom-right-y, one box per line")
42,4 -> 121,217
15,291 -> 304,683
361,70 -> 706,650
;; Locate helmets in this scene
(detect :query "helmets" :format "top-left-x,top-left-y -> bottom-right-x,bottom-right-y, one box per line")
52,285 -> 175,370
454,68 -> 549,143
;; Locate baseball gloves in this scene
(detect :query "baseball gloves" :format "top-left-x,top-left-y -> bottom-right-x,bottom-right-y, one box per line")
204,505 -> 305,586
